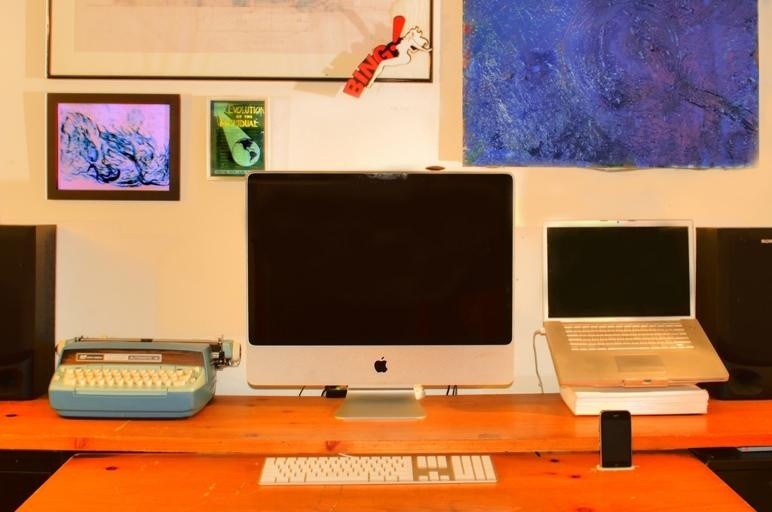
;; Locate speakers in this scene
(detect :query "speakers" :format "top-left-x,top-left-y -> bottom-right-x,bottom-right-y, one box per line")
696,226 -> 772,400
0,226 -> 55,400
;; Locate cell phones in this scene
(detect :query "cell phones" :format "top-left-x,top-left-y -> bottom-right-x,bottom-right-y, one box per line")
600,410 -> 632,467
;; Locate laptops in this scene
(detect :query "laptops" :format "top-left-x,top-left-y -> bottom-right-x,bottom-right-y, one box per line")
542,218 -> 729,387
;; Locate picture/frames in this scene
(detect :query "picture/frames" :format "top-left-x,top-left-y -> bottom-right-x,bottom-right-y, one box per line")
206,96 -> 271,180
47,0 -> 433,83
45,93 -> 179,202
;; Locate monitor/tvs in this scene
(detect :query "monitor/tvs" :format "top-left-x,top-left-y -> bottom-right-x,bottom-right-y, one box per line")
246,171 -> 516,420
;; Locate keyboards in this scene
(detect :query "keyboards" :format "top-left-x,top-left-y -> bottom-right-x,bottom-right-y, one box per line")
259,454 -> 498,485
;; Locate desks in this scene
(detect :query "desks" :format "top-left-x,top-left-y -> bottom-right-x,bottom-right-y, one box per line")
0,397 -> 772,512
